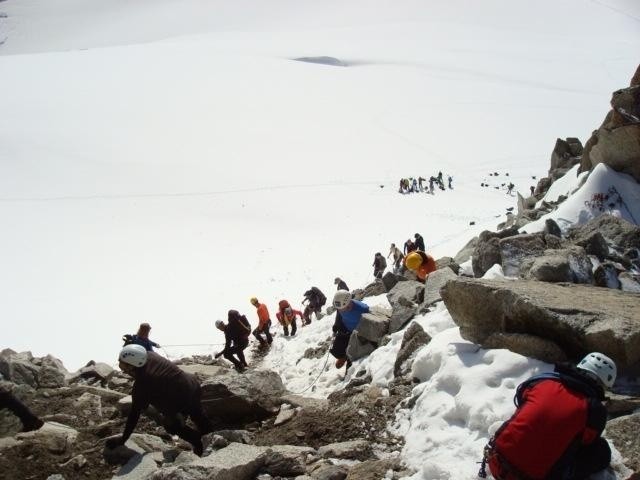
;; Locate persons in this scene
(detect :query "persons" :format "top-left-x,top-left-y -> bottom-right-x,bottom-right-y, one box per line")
529,185 -> 536,196
249,295 -> 273,349
333,278 -> 350,291
372,232 -> 426,279
397,251 -> 437,304
122,322 -> 162,353
398,170 -> 453,195
474,348 -> 617,479
276,307 -> 307,336
330,289 -> 371,382
106,345 -> 203,457
302,290 -> 322,324
505,182 -> 515,194
213,319 -> 249,371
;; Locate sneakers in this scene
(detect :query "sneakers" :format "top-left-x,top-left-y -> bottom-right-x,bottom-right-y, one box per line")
336,356 -> 347,368
190,429 -> 203,457
16,419 -> 45,433
235,361 -> 247,371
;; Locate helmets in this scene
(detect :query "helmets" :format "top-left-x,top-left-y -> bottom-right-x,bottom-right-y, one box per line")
576,352 -> 617,388
118,343 -> 148,368
333,289 -> 352,309
406,253 -> 423,270
215,320 -> 223,327
251,297 -> 257,305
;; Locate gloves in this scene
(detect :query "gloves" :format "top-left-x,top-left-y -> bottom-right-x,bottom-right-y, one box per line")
105,436 -> 126,450
